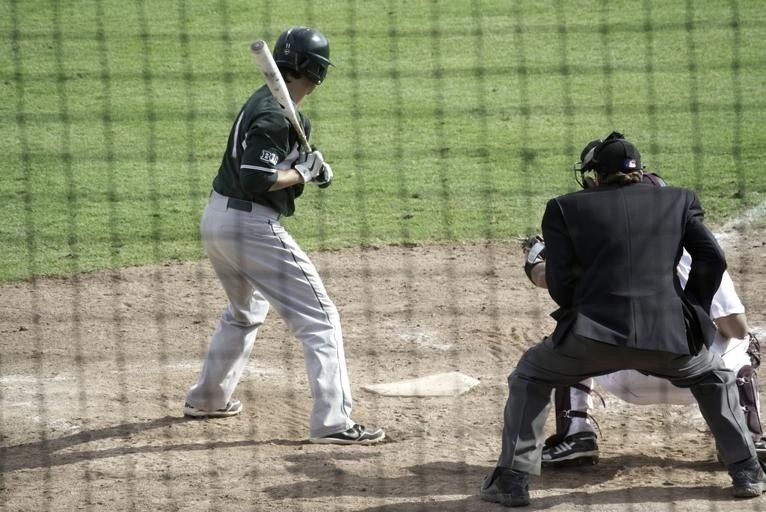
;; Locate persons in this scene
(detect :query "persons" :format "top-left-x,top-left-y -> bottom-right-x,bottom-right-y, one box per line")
184,27 -> 386,445
482,132 -> 766,507
521,172 -> 765,467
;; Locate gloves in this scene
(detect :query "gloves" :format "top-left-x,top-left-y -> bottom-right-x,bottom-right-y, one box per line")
293,143 -> 334,188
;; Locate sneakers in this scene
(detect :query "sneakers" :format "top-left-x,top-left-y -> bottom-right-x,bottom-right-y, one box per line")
310,423 -> 386,446
726,439 -> 765,497
541,432 -> 600,467
183,396 -> 243,418
479,466 -> 531,507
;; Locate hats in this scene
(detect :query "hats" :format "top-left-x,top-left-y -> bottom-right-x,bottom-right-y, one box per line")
581,131 -> 642,175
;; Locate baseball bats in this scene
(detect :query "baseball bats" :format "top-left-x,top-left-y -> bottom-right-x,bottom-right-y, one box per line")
251,40 -> 330,188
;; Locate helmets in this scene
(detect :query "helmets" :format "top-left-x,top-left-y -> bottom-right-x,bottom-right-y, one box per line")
271,26 -> 336,85
